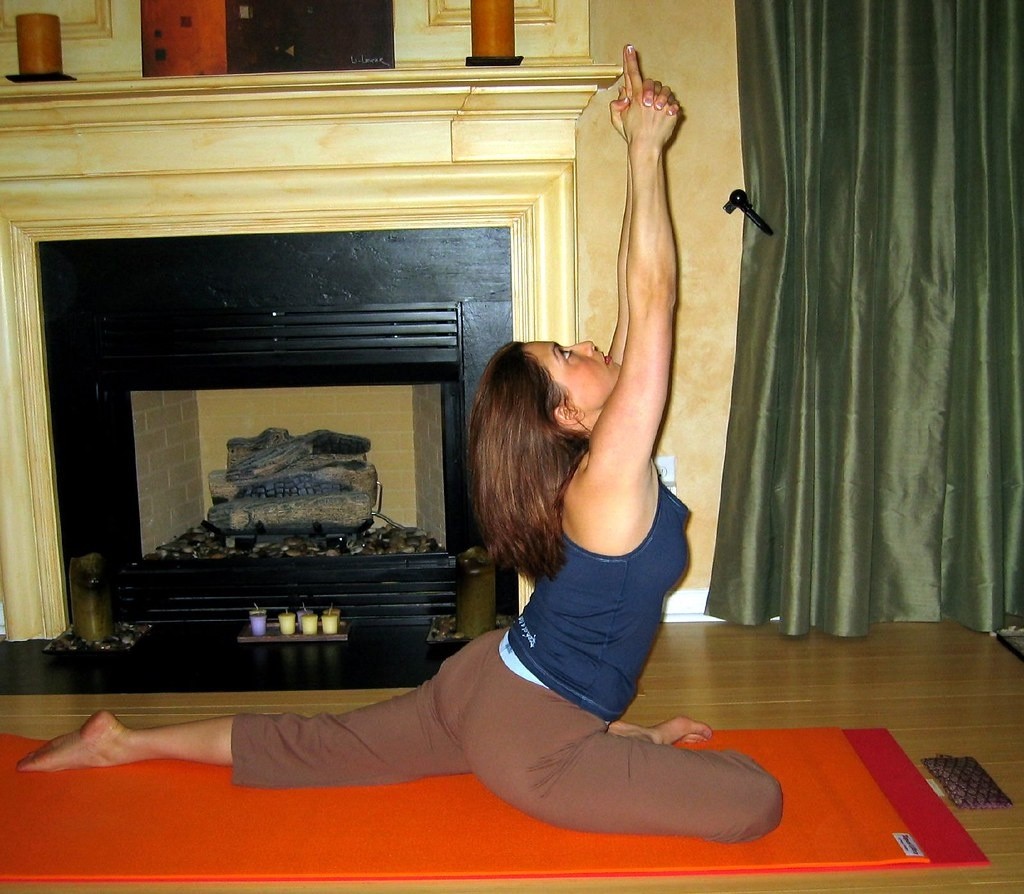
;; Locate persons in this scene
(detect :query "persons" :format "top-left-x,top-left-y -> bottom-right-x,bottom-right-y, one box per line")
15,43 -> 783,840
